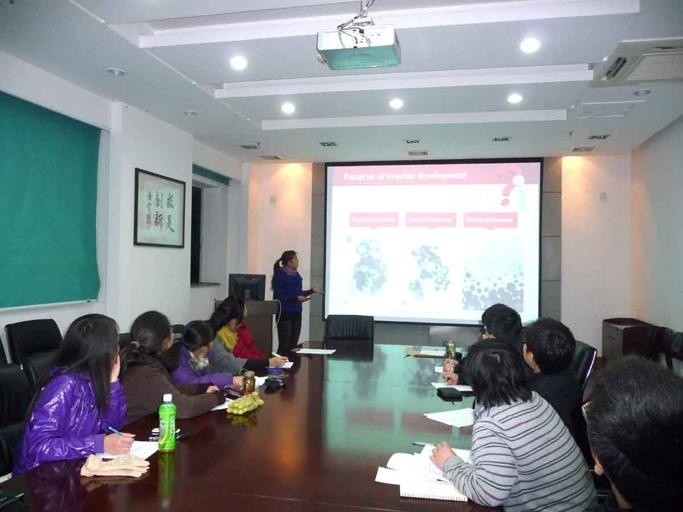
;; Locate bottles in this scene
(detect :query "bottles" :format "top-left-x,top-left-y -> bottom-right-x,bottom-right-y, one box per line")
243,370 -> 255,394
159,394 -> 176,452
446,343 -> 455,358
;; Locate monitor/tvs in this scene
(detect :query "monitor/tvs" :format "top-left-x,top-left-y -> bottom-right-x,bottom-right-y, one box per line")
228,272 -> 265,302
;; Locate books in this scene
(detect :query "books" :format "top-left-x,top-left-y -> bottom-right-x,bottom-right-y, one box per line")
399,442 -> 472,503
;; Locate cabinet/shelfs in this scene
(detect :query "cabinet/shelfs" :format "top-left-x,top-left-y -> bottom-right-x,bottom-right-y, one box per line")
603,317 -> 654,363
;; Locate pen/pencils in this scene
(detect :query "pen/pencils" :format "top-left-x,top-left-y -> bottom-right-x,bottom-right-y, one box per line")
108,426 -> 123,433
414,440 -> 437,447
271,352 -> 289,362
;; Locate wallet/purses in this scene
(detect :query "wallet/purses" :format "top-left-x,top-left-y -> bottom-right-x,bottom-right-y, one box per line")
437,387 -> 462,406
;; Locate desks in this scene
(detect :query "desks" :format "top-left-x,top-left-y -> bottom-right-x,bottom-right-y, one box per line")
0,340 -> 511,511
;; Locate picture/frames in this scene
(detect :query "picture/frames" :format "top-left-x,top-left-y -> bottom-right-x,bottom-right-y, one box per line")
133,166 -> 186,249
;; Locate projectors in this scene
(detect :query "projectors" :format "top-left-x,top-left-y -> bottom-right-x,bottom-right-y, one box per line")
315,21 -> 402,71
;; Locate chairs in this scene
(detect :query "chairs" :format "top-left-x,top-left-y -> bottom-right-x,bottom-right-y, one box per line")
23,350 -> 61,395
0,364 -> 37,477
565,340 -> 598,390
325,314 -> 375,341
6,318 -> 65,363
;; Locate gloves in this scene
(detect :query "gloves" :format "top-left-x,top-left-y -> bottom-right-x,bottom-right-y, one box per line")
80,455 -> 150,478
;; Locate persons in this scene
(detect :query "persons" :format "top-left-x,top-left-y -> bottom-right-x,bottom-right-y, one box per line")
206,303 -> 287,373
118,310 -> 226,429
441,304 -> 533,385
272,250 -> 325,359
162,321 -> 245,393
228,294 -> 278,361
518,317 -> 613,489
430,339 -> 600,512
579,355 -> 683,512
11,314 -> 135,480
